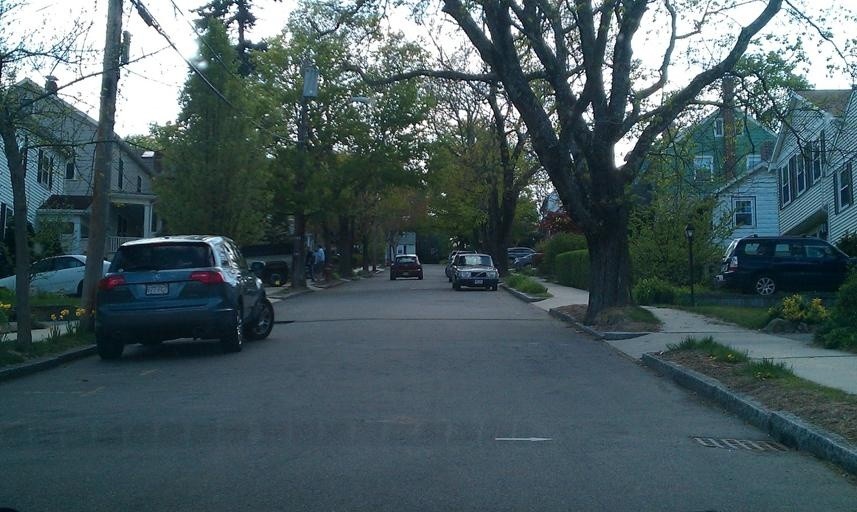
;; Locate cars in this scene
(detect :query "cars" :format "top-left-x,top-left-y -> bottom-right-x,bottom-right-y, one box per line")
390,253 -> 423,280
0,235 -> 274,359
445,247 -> 545,291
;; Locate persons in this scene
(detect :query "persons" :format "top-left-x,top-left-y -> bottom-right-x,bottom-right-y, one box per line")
316,244 -> 325,279
305,245 -> 315,283
313,250 -> 319,280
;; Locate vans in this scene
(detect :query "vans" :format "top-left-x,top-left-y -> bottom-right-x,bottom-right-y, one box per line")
714,233 -> 854,296
240,243 -> 292,287
